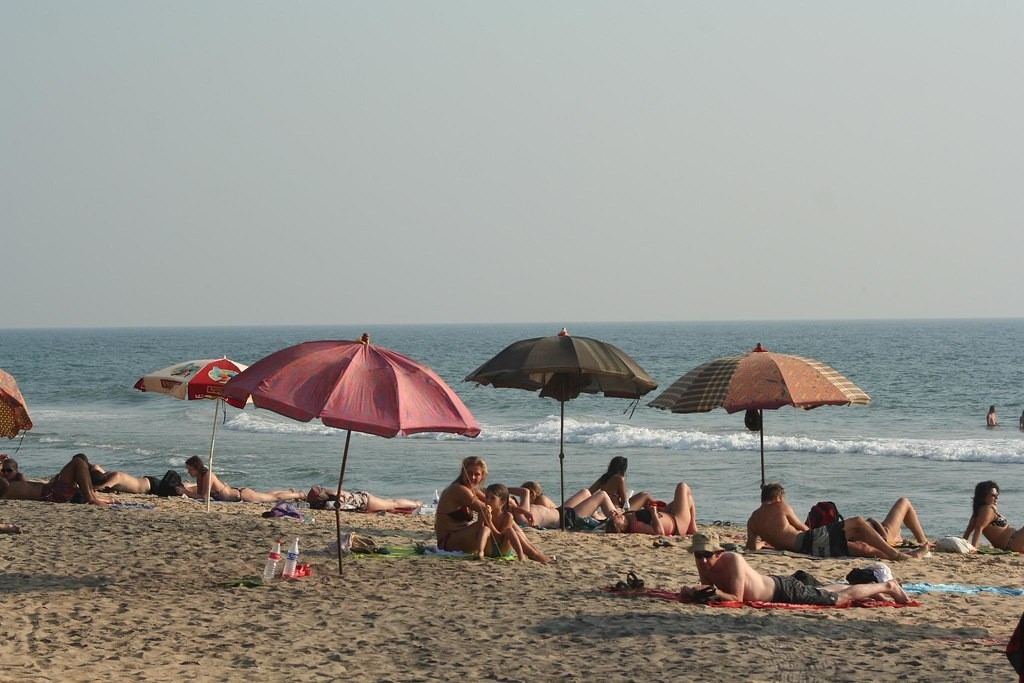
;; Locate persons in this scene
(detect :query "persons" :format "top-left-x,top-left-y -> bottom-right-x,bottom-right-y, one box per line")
521,481 -> 556,508
308,487 -> 423,511
478,483 -> 526,562
588,456 -> 656,510
508,486 -> 617,527
177,456 -> 307,501
91,470 -> 197,493
987,406 -> 999,426
435,456 -> 556,563
1020,410 -> 1024,427
746,484 -> 929,562
1006,612 -> 1024,683
963,482 -> 1024,553
615,481 -> 698,536
74,453 -> 105,474
867,498 -> 937,547
681,529 -> 911,605
2,459 -> 24,481
0,457 -> 115,504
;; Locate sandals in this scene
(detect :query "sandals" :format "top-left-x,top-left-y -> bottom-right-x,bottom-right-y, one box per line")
681,584 -> 721,604
609,573 -> 644,593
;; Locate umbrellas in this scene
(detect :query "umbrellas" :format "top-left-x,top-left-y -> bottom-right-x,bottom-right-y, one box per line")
136,355 -> 253,511
649,342 -> 871,484
0,367 -> 33,438
225,333 -> 481,574
462,327 -> 658,534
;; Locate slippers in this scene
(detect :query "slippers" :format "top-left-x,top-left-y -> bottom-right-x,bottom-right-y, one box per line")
893,540 -> 918,549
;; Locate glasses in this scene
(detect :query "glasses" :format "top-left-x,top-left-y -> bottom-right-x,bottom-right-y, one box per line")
988,493 -> 999,498
694,551 -> 715,560
1,468 -> 17,474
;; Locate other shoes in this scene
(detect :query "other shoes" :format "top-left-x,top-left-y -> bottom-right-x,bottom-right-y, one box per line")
653,538 -> 675,547
350,535 -> 390,555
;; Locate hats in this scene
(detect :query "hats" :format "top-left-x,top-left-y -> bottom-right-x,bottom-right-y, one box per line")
306,487 -> 324,505
646,500 -> 667,512
686,530 -> 725,554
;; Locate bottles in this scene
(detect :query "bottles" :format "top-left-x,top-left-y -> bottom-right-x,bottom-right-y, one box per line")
342,521 -> 350,544
433,490 -> 439,508
283,537 -> 299,577
263,540 -> 282,579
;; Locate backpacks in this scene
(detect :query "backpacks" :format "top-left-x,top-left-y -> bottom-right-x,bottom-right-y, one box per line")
805,501 -> 846,529
